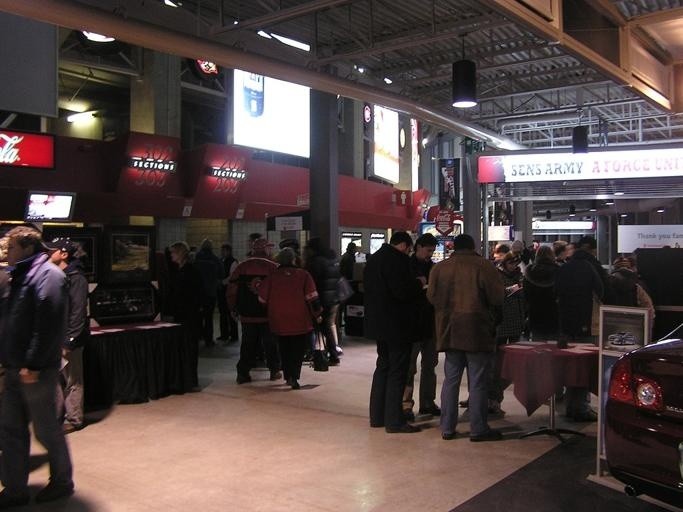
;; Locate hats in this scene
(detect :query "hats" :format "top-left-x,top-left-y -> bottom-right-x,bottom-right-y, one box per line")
252,238 -> 274,250
42,237 -> 72,252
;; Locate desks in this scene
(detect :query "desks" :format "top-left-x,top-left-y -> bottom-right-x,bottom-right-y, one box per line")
497,340 -> 600,445
83,318 -> 197,404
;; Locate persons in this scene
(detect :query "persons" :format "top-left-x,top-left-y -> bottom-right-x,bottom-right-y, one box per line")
40,238 -> 90,433
0,223 -> 76,507
409,233 -> 442,423
555,236 -> 598,422
362,232 -> 428,433
161,233 -> 354,390
487,240 -> 562,418
340,242 -> 358,327
427,234 -> 500,442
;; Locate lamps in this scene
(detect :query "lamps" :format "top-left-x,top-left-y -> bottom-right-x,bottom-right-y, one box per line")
571,106 -> 589,154
545,190 -> 613,222
449,31 -> 478,110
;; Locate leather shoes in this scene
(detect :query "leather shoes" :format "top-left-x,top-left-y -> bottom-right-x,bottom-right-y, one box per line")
385,404 -> 441,433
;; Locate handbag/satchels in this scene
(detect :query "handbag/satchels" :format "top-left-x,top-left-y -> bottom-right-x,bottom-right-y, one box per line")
338,276 -> 354,301
314,349 -> 328,372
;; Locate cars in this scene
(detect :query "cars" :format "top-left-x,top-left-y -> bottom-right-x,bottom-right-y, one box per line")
602,318 -> 683,512
607,329 -> 636,347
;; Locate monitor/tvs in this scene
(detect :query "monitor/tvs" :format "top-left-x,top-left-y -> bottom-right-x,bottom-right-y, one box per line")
24,189 -> 77,222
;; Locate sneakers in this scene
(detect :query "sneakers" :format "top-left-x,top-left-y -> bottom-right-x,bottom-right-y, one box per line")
472,431 -> 501,441
